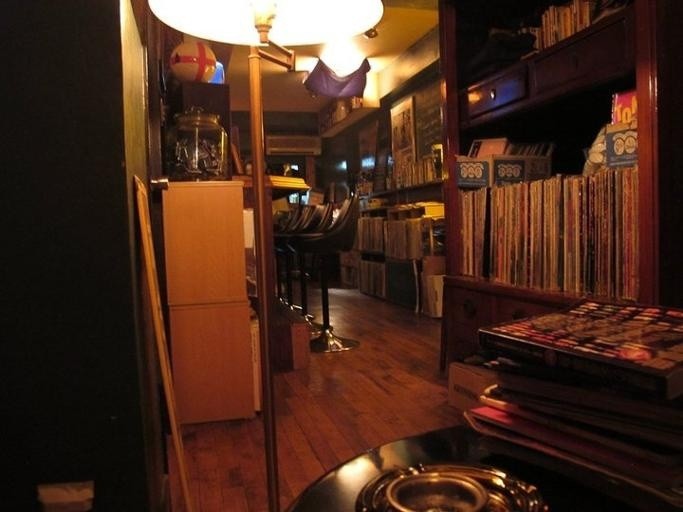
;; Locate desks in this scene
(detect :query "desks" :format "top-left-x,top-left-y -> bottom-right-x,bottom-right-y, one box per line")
280,423 -> 682,512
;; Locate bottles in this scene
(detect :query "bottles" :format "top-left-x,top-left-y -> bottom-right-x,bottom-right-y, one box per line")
165,107 -> 231,182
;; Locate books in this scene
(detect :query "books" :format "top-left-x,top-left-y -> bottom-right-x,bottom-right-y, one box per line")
511,0 -> 631,62
338,155 -> 443,316
451,139 -> 638,301
466,297 -> 683,508
